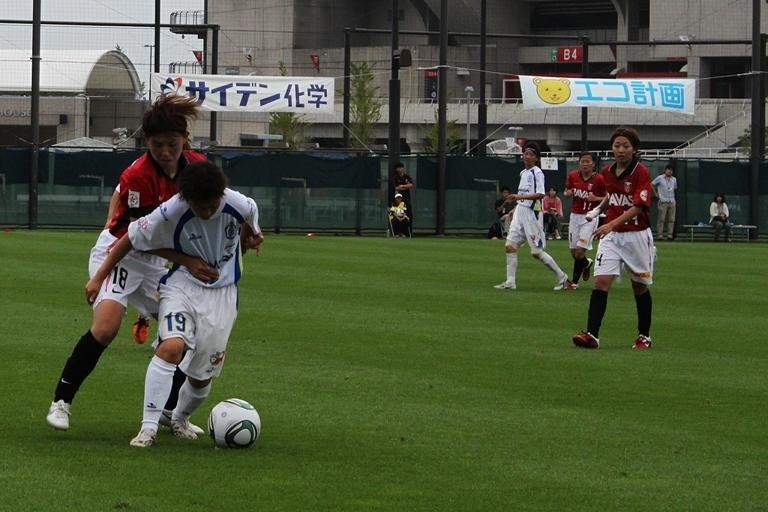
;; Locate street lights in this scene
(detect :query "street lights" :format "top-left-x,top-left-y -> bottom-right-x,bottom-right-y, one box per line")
463,85 -> 475,155
77,94 -> 90,139
508,125 -> 524,142
386,45 -> 412,210
142,44 -> 156,100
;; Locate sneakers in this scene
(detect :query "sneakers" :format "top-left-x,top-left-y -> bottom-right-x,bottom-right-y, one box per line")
632,334 -> 651,350
553,257 -> 593,291
572,330 -> 600,348
133,312 -> 150,344
493,281 -> 516,289
130,408 -> 204,448
46,399 -> 72,430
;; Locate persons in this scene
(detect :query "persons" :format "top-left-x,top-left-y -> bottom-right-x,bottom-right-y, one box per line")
563,151 -> 606,292
650,164 -> 679,240
542,188 -> 564,239
572,128 -> 654,348
709,192 -> 732,242
495,187 -> 515,239
491,142 -> 567,292
46,93 -> 264,429
84,160 -> 260,446
391,163 -> 413,236
388,194 -> 410,238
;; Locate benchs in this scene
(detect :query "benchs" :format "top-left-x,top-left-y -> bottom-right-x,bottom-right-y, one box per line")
682,224 -> 757,243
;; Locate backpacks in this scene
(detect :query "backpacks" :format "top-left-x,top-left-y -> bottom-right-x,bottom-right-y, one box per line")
487,221 -> 504,239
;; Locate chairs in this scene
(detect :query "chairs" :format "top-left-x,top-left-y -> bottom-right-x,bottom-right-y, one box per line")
498,219 -> 507,239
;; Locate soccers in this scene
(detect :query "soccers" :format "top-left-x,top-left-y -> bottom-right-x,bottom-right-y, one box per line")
205,397 -> 261,447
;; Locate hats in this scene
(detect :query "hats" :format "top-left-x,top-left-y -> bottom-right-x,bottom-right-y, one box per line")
395,193 -> 402,198
